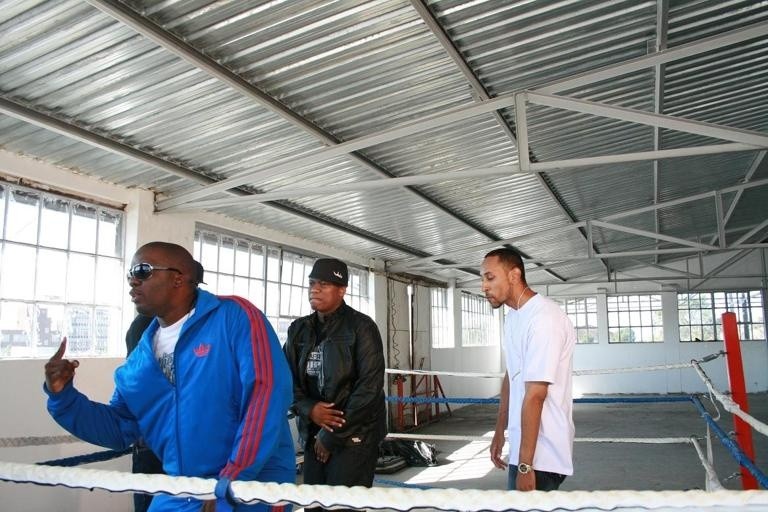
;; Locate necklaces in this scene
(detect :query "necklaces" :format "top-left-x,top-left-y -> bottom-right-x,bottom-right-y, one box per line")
154,307 -> 195,354
517,286 -> 529,310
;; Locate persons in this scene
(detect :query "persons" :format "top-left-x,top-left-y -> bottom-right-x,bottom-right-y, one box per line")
124,258 -> 208,512
479,248 -> 576,491
281,258 -> 388,511
42,240 -> 298,511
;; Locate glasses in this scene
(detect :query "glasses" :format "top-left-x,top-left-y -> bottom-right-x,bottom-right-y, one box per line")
126,263 -> 183,279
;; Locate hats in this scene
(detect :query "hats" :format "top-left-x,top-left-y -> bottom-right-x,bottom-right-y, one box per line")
308,257 -> 349,287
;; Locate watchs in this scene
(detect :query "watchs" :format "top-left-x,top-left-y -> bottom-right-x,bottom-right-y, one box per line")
518,462 -> 533,475
